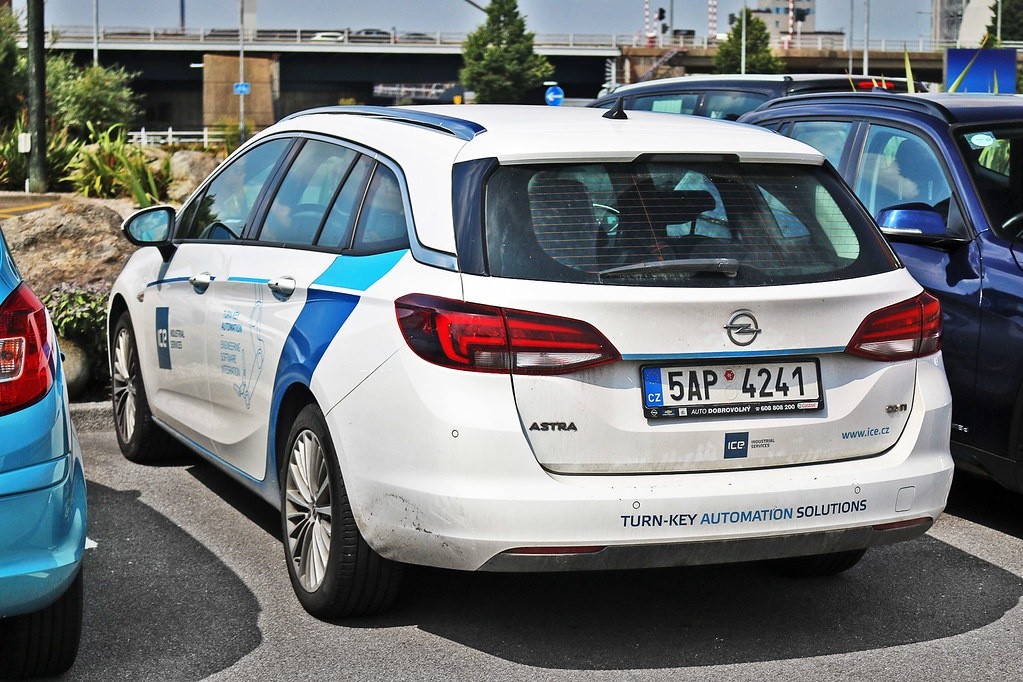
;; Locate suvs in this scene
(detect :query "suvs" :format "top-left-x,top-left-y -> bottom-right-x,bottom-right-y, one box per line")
575,71 -> 933,125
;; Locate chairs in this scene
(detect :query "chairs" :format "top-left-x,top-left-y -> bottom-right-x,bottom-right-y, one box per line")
530,180 -> 599,273
880,137 -> 949,228
639,190 -> 748,264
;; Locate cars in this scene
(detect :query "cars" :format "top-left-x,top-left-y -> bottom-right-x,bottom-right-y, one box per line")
658,87 -> 1022,501
398,31 -> 435,41
103,102 -> 955,625
0,226 -> 90,681
355,28 -> 390,37
309,31 -> 342,43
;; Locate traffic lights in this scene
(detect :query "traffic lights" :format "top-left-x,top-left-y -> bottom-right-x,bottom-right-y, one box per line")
657,8 -> 667,20
661,23 -> 669,34
